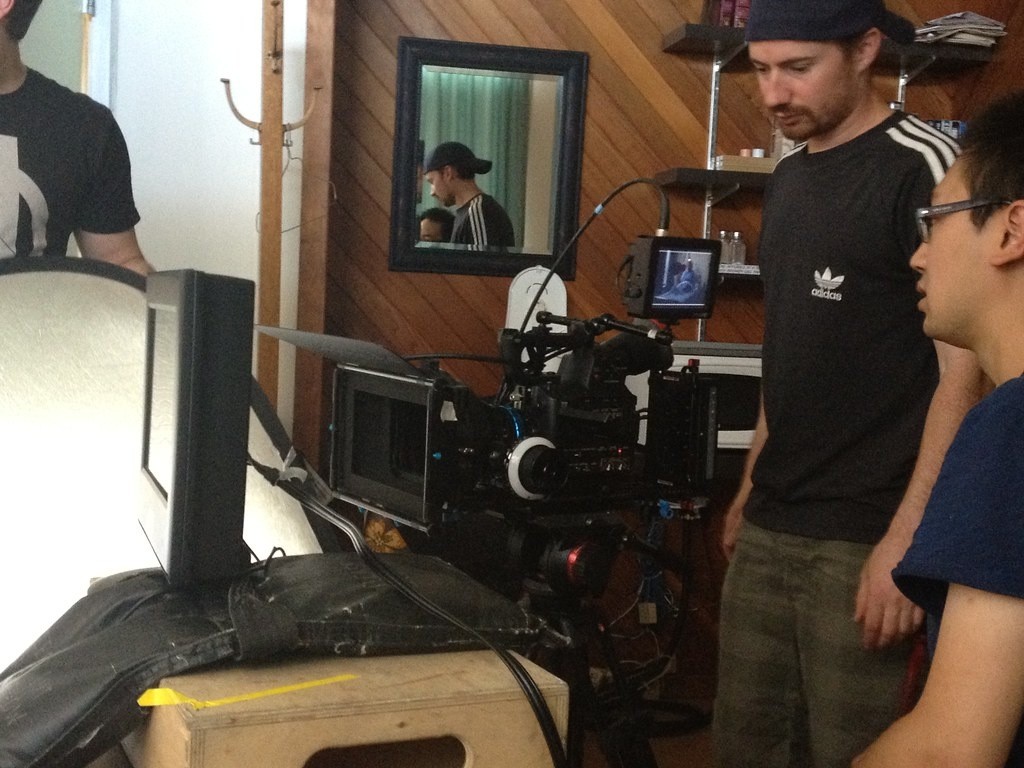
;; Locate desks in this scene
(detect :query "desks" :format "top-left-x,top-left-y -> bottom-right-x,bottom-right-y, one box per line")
88,574 -> 571,768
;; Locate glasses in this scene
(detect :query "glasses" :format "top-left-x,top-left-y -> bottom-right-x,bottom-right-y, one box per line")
914,193 -> 1024,243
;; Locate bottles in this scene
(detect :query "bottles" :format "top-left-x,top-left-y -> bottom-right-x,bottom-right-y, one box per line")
729,232 -> 745,266
717,231 -> 729,263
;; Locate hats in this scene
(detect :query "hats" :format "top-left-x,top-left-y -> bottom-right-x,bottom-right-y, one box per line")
745,0 -> 915,46
422,142 -> 493,175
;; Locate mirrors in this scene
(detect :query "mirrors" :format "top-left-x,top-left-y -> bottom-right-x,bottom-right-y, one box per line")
388,36 -> 590,282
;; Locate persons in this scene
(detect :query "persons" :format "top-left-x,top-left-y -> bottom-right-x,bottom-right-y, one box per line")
851,85 -> 1024,768
419,206 -> 455,243
709,1 -> 965,768
681,261 -> 695,282
0,0 -> 159,278
423,141 -> 516,245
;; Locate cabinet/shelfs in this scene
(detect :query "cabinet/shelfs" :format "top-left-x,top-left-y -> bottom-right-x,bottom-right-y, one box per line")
651,21 -> 992,359
